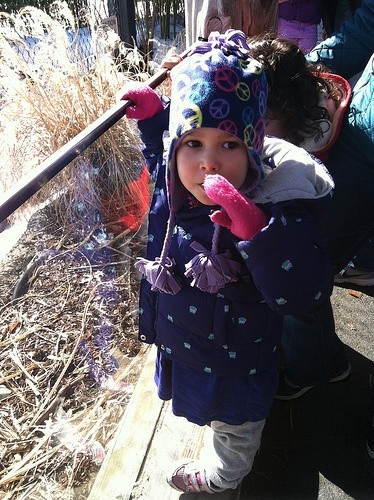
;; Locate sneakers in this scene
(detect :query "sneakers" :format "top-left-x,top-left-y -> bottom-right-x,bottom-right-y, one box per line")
166,457 -> 226,494
333,256 -> 374,286
273,350 -> 351,401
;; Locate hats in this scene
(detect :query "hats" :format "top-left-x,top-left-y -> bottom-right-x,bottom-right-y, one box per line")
133,29 -> 270,295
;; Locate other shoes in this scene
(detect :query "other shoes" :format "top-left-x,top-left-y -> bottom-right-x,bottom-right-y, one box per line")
366,387 -> 374,459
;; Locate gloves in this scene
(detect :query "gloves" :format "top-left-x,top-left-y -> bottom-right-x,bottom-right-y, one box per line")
117,80 -> 165,121
204,173 -> 267,241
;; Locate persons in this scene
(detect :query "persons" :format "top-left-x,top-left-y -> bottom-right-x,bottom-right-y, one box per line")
243,33 -> 374,402
274,0 -> 374,287
116,28 -> 336,495
